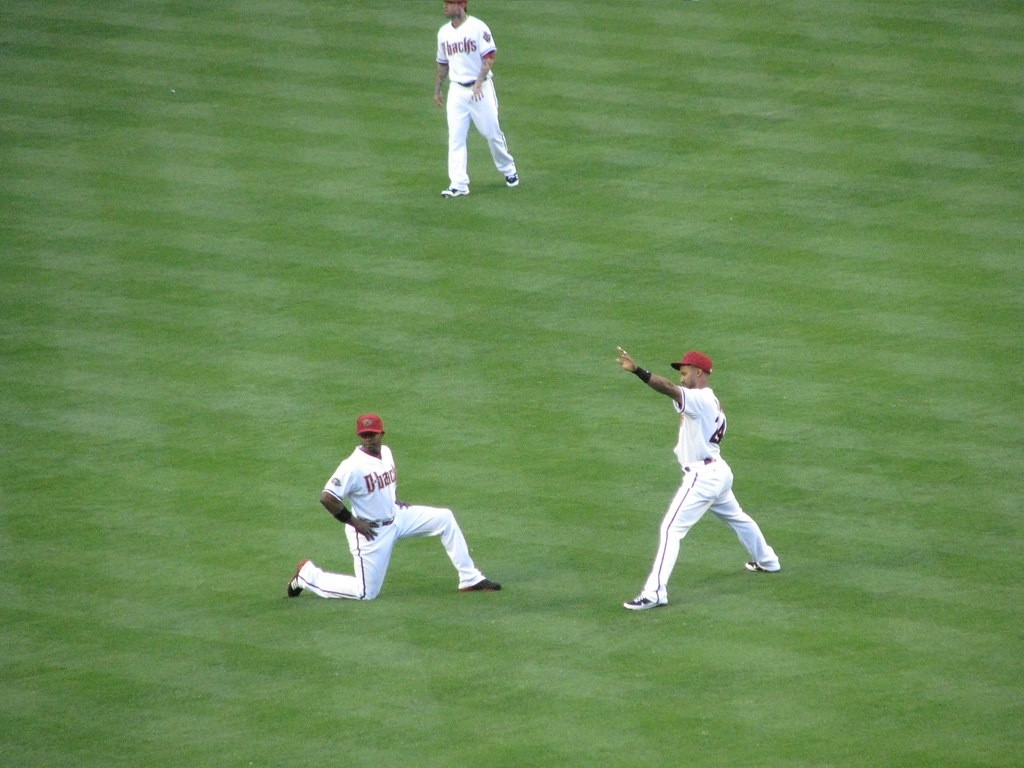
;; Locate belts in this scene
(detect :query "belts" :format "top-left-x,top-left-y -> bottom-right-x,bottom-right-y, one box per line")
357,517 -> 395,527
683,457 -> 713,470
458,79 -> 487,87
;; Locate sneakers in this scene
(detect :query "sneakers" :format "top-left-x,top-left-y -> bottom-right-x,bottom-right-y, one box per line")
461,580 -> 501,591
505,173 -> 519,187
624,594 -> 668,610
288,560 -> 308,598
442,186 -> 470,197
745,562 -> 781,572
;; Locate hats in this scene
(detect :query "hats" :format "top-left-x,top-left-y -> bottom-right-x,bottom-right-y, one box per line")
671,351 -> 713,373
356,414 -> 384,434
444,0 -> 467,3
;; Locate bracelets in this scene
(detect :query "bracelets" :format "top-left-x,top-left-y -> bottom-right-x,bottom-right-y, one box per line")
334,507 -> 353,523
634,366 -> 652,384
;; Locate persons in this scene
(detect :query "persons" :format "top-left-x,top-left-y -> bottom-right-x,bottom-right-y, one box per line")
613,344 -> 781,611
433,1 -> 521,198
286,413 -> 503,600
